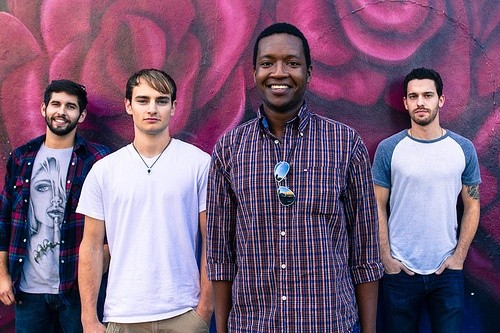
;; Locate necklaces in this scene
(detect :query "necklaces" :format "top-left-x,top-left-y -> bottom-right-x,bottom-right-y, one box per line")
132,140 -> 172,174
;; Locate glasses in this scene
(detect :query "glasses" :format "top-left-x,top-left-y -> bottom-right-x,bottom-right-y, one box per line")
274,161 -> 296,206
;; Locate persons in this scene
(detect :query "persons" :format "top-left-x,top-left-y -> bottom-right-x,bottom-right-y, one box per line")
371,67 -> 483,333
0,79 -> 112,333
205,23 -> 384,333
78,68 -> 211,333
